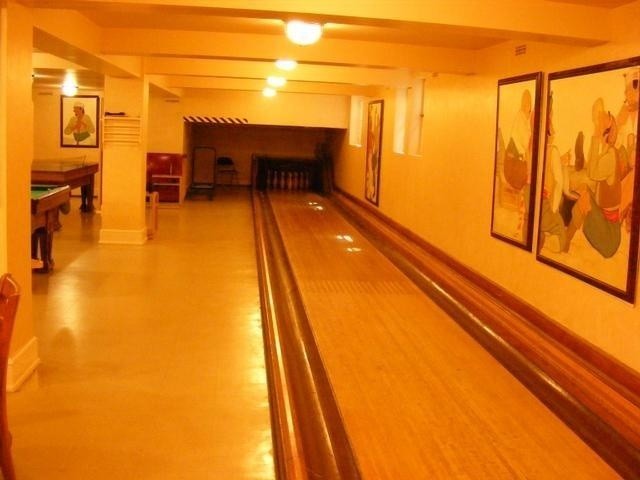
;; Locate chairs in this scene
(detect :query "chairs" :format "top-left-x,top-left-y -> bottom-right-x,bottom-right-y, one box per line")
216,156 -> 240,190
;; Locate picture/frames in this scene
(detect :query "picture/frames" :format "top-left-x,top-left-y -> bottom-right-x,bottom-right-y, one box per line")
490,56 -> 639,306
365,100 -> 384,206
60,95 -> 99,148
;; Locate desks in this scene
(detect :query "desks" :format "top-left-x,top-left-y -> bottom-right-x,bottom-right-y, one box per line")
30,156 -> 100,276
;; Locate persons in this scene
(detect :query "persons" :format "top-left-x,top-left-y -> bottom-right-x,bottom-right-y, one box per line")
64,101 -> 95,145
541,68 -> 640,258
504,88 -> 535,190
